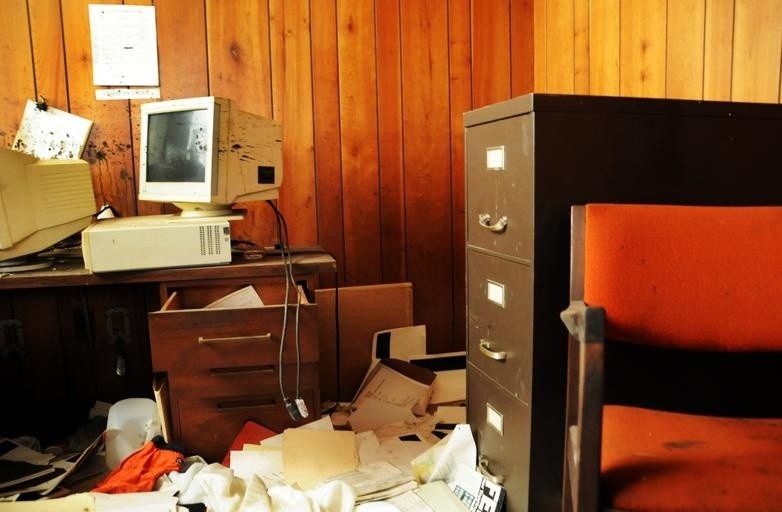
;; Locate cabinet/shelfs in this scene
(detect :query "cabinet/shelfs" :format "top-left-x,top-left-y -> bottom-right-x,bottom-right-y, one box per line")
465,94 -> 782,511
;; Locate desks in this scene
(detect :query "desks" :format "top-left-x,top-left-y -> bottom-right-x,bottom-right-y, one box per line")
1,243 -> 336,464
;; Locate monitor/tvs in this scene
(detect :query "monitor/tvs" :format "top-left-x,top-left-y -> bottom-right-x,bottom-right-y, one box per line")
139,96 -> 282,218
0,148 -> 98,273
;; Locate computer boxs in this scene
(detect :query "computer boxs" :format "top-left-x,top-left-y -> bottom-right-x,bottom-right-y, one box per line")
82,214 -> 232,274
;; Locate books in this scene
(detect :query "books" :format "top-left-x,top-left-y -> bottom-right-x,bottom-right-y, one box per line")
445,467 -> 508,511
2,458 -> 55,491
11,98 -> 93,161
352,356 -> 436,413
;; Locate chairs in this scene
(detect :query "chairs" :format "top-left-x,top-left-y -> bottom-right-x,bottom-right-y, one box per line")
563,195 -> 782,511
